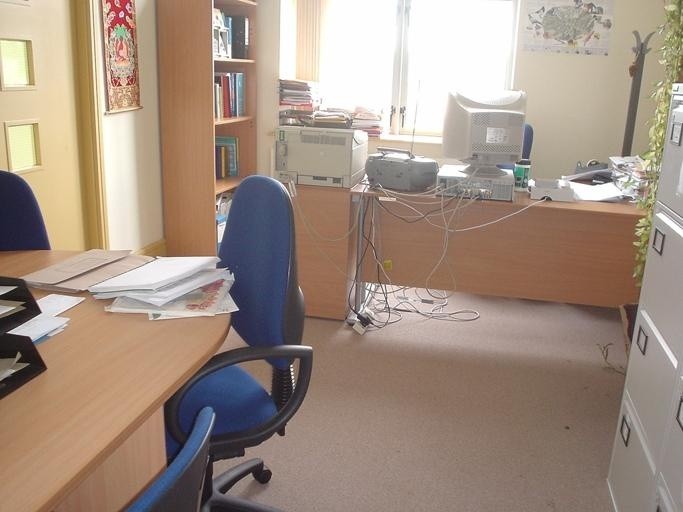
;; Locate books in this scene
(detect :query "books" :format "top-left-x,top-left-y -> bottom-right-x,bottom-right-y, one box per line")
216,136 -> 240,178
215,71 -> 246,120
224,16 -> 233,58
18,246 -> 236,316
279,79 -> 384,138
232,15 -> 250,59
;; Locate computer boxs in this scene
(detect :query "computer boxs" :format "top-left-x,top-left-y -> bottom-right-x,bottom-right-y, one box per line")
436,164 -> 515,203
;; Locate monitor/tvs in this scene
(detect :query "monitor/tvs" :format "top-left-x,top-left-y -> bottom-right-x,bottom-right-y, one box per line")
441,85 -> 528,177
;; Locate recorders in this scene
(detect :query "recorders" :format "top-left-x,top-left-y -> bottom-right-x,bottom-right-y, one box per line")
365,147 -> 439,192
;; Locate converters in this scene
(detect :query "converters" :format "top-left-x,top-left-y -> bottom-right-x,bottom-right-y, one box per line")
357,313 -> 370,326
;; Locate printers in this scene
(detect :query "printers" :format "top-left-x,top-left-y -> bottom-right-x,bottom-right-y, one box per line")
270,125 -> 368,189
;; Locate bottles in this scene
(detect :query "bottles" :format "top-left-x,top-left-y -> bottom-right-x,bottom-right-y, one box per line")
513,159 -> 531,192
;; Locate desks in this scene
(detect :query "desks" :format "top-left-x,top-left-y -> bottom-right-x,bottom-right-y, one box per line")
0,248 -> 233,512
280,179 -> 648,322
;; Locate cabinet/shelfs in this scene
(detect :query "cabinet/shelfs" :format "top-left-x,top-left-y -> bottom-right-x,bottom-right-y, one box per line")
606,81 -> 683,512
156,0 -> 257,257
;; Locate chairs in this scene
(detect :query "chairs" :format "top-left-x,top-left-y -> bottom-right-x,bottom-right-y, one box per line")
164,175 -> 314,512
126,407 -> 217,512
498,124 -> 534,168
0,169 -> 51,251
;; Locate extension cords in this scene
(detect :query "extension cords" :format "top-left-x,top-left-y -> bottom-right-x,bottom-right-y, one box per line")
352,306 -> 375,335
